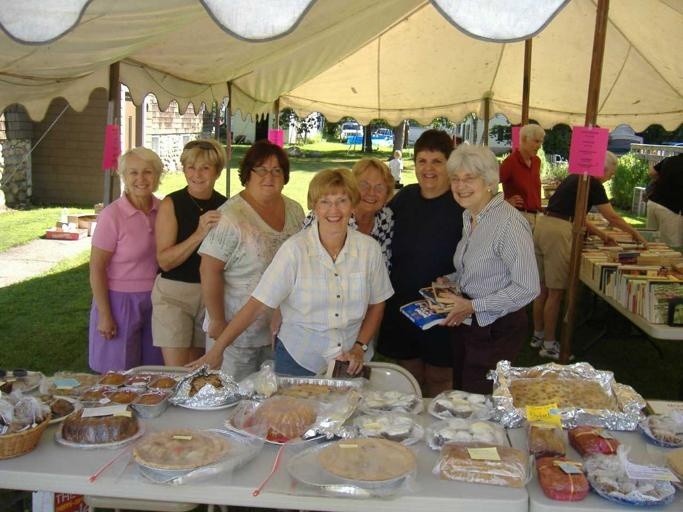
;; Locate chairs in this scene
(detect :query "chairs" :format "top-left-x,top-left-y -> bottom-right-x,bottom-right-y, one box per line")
351,362 -> 423,399
78,363 -> 232,511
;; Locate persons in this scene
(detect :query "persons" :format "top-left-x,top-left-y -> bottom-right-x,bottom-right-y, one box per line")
268,155 -> 395,362
87,147 -> 164,375
181,167 -> 396,377
149,138 -> 231,366
196,142 -> 305,384
388,149 -> 404,189
384,129 -> 467,402
431,143 -> 541,394
645,153 -> 683,249
531,150 -> 649,361
497,126 -> 543,235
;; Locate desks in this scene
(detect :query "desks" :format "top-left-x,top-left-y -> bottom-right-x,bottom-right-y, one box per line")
539,198 -> 682,341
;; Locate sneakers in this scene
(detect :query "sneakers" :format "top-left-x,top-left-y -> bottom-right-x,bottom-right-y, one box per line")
539,341 -> 575,362
529,336 -> 544,348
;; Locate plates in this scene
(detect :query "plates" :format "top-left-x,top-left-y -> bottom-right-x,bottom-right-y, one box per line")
39,384 -> 83,396
399,420 -> 424,447
359,397 -> 424,418
425,419 -> 503,452
427,401 -> 494,420
41,396 -> 82,424
167,396 -> 243,412
313,441 -> 415,483
0,380 -> 39,394
133,430 -> 229,476
54,417 -> 145,448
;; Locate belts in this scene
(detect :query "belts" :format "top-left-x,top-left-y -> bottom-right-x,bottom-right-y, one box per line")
516,207 -> 538,214
544,210 -> 573,222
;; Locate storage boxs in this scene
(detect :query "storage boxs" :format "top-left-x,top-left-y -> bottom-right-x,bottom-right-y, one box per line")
46,214 -> 102,240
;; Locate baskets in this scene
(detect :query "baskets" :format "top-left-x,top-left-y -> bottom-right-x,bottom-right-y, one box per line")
1,412 -> 51,460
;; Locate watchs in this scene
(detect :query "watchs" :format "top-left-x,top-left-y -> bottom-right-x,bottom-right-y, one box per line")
355,341 -> 367,353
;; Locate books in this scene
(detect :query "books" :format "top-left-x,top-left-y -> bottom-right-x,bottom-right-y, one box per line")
579,211 -> 683,324
399,282 -> 473,331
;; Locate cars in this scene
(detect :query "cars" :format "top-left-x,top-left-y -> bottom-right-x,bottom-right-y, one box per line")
371,126 -> 391,137
340,121 -> 362,141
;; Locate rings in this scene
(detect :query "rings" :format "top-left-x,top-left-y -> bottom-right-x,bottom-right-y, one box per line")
101,334 -> 105,337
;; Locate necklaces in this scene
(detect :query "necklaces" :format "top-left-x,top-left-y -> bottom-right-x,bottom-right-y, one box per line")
187,186 -> 215,213
319,238 -> 346,263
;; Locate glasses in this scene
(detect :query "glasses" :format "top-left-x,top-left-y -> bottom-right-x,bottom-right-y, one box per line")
356,181 -> 387,194
251,165 -> 285,178
183,140 -> 218,157
318,197 -> 351,209
447,174 -> 479,187
